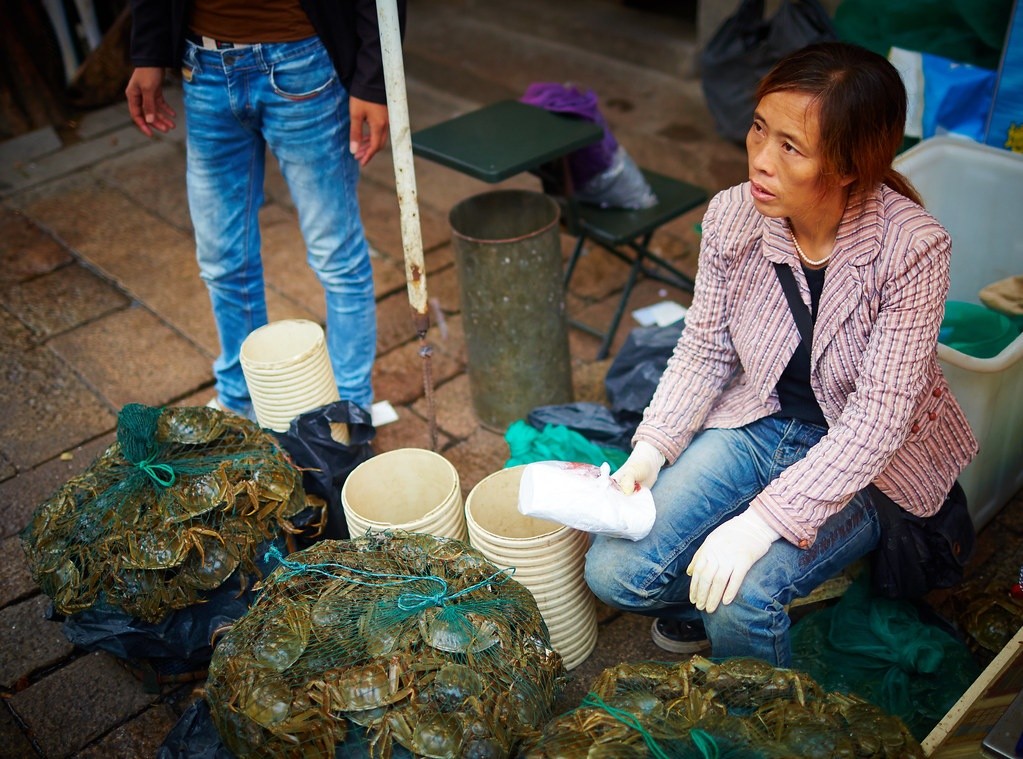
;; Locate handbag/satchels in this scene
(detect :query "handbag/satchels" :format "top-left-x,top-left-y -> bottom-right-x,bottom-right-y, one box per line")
695,0 -> 837,147
520,80 -> 660,209
264,400 -> 377,541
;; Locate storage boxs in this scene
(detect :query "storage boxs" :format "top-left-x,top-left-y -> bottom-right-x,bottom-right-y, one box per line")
887,131 -> 1023,536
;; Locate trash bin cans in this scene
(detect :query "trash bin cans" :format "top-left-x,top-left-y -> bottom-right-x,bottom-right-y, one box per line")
446,187 -> 576,438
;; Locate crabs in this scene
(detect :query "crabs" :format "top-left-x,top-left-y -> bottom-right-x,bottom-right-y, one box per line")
32,401 -> 921,758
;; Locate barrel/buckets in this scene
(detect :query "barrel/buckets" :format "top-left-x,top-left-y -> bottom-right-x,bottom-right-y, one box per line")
448,189 -> 573,435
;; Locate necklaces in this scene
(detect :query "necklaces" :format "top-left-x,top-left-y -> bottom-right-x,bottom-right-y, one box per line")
786,218 -> 835,265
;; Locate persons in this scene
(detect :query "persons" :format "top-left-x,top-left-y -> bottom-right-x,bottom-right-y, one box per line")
126,0 -> 407,445
586,41 -> 980,669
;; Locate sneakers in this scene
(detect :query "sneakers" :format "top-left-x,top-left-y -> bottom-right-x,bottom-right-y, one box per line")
650,612 -> 712,652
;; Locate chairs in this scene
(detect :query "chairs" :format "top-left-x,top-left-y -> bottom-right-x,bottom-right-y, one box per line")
407,100 -> 708,362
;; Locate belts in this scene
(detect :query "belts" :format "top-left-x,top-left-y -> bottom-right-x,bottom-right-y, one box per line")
186,36 -> 234,51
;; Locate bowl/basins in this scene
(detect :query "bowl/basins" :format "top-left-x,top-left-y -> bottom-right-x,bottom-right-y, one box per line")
239,318 -> 341,434
341,448 -> 599,673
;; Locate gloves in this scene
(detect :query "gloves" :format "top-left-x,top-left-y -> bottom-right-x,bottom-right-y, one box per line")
611,437 -> 668,495
686,506 -> 782,614
